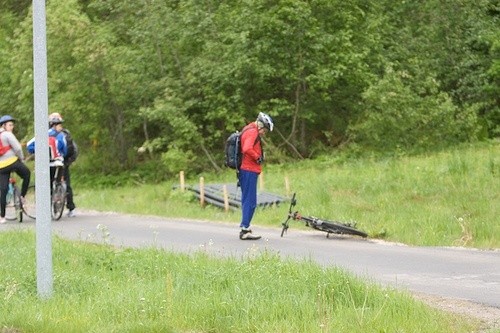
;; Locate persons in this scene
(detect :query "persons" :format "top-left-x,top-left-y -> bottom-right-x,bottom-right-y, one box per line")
239,112 -> 273,240
0,115 -> 30,224
27,112 -> 77,217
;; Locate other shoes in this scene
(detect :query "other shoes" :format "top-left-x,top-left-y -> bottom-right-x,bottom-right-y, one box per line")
0,216 -> 6,223
67,208 -> 77,217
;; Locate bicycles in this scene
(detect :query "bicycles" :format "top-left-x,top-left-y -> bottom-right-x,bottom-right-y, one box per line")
20,156 -> 67,221
280,194 -> 368,240
3,169 -> 24,223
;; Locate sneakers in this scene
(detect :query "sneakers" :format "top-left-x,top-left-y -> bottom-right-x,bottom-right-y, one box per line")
240,225 -> 261,240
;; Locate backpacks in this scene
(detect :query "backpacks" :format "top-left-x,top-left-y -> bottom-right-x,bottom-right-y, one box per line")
223,126 -> 264,178
48,130 -> 64,161
0,129 -> 12,156
63,128 -> 79,163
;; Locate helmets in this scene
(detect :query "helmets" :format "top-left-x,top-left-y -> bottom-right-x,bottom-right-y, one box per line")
259,111 -> 274,132
0,115 -> 16,123
49,112 -> 64,123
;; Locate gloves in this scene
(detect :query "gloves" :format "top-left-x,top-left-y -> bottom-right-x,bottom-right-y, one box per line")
257,157 -> 261,164
